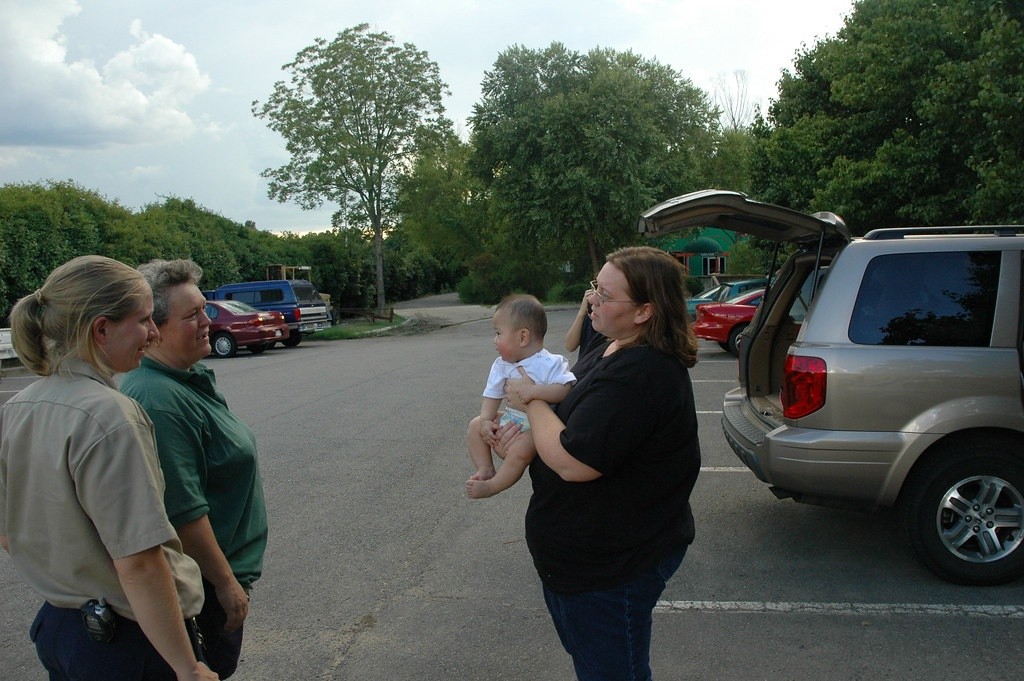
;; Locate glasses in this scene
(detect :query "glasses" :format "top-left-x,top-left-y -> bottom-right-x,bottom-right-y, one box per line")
589,280 -> 641,302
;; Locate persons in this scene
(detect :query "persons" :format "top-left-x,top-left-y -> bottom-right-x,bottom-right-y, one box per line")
119,259 -> 269,681
504,245 -> 701,681
566,289 -> 611,361
465,293 -> 578,499
0,254 -> 220,681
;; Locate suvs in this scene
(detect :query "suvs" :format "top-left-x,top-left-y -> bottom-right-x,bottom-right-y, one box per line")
637,187 -> 1024,586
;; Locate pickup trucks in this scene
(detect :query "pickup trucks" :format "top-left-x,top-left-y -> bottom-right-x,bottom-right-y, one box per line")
686,279 -> 770,321
199,280 -> 331,348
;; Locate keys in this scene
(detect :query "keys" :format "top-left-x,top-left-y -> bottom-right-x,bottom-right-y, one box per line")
197,632 -> 207,650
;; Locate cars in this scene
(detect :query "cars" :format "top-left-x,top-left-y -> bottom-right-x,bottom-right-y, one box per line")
691,285 -> 768,357
205,300 -> 290,357
